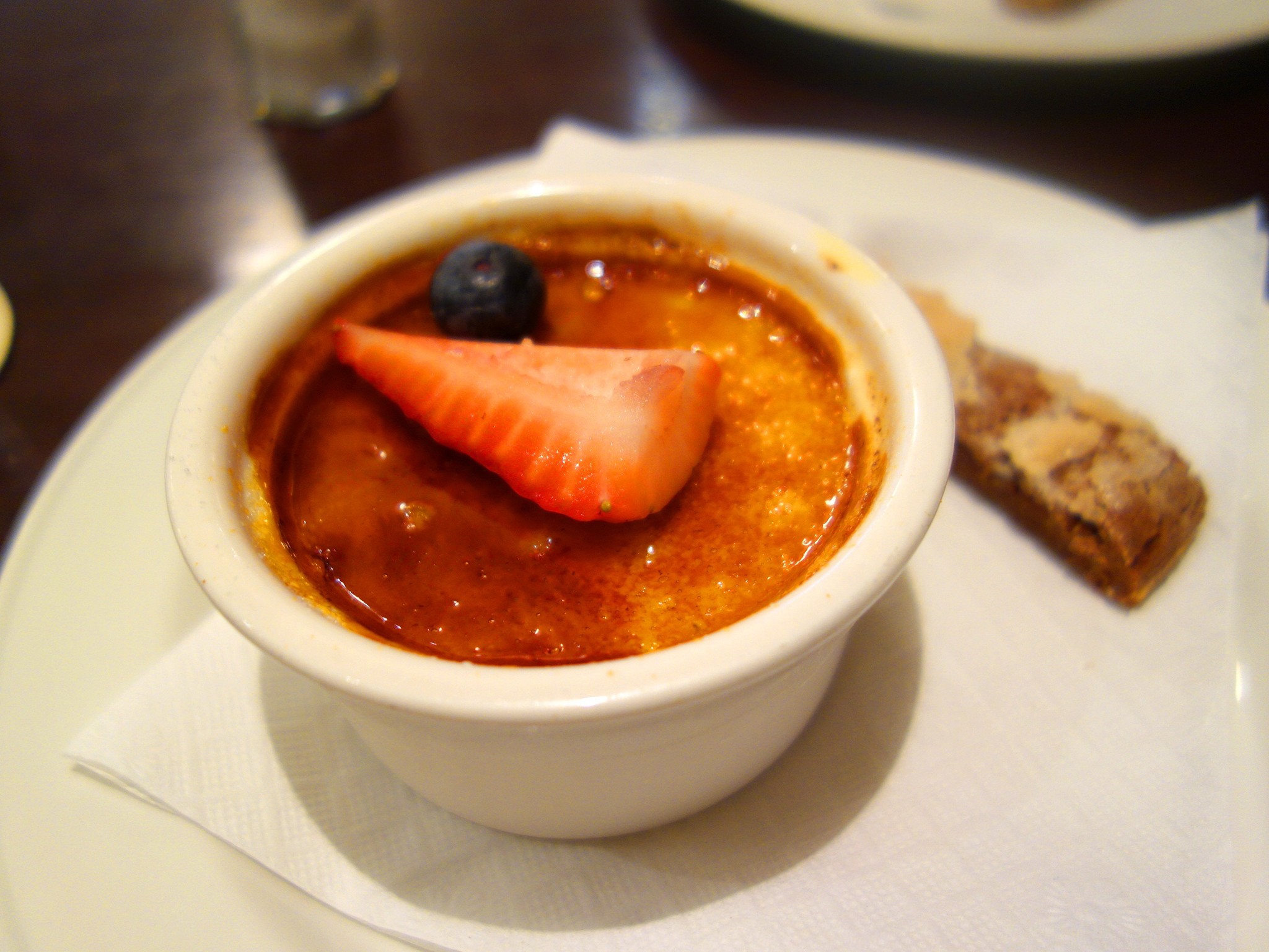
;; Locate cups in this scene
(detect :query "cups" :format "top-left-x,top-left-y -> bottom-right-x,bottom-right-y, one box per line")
163,152 -> 956,844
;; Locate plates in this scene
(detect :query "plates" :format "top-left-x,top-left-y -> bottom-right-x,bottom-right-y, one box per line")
0,134 -> 1268,951
738,0 -> 1269,60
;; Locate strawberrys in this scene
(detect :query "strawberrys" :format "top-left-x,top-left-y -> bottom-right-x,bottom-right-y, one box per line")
327,320 -> 723,521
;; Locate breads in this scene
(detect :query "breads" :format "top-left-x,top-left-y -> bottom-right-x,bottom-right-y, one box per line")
906,284 -> 1206,611
